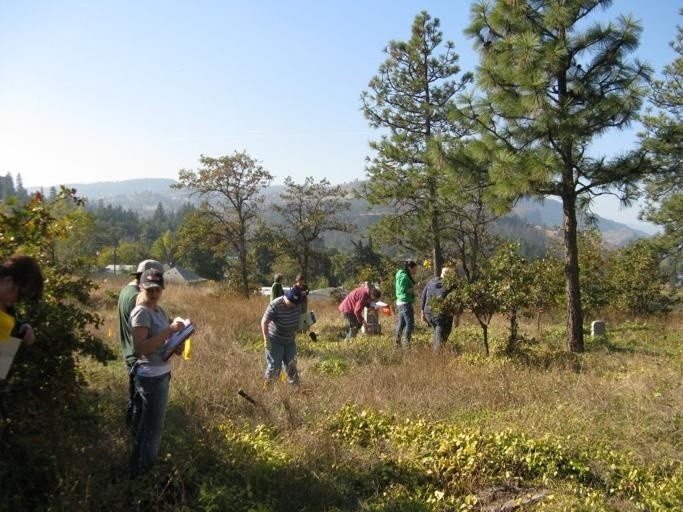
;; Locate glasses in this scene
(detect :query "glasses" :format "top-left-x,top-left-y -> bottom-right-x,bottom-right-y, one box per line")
16,288 -> 28,304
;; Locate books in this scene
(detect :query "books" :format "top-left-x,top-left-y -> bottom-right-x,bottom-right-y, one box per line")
163,317 -> 194,362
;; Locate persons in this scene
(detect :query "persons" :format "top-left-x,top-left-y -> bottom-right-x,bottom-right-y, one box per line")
336,285 -> 381,341
125,267 -> 187,480
116,258 -> 164,442
0,250 -> 43,385
419,267 -> 461,353
291,272 -> 308,315
392,259 -> 418,349
269,272 -> 284,302
258,286 -> 304,394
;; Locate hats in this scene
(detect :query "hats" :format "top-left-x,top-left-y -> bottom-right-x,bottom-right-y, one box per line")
129,260 -> 165,290
284,286 -> 305,305
